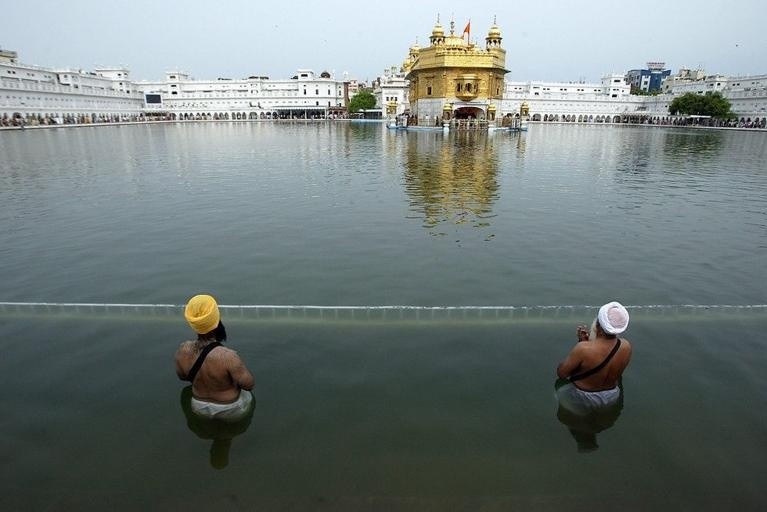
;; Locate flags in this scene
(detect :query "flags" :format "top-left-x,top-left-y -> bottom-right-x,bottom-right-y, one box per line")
462,18 -> 472,34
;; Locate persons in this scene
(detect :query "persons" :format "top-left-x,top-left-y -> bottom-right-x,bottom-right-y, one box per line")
172,294 -> 258,424
554,302 -> 633,417
